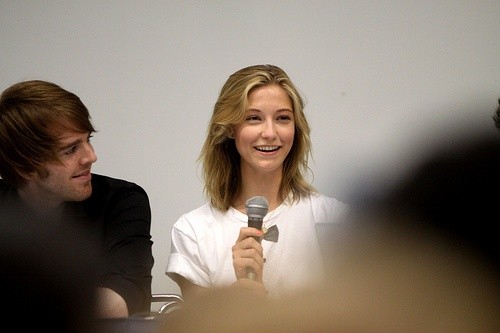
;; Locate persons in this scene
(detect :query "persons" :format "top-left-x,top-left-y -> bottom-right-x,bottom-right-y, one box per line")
165,64 -> 350,303
153,132 -> 500,333
0,80 -> 154,333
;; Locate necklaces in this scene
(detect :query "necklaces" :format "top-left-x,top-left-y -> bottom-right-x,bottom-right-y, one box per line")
262,210 -> 275,233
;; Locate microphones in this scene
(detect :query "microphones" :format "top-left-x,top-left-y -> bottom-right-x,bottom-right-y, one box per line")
244,195 -> 269,281
